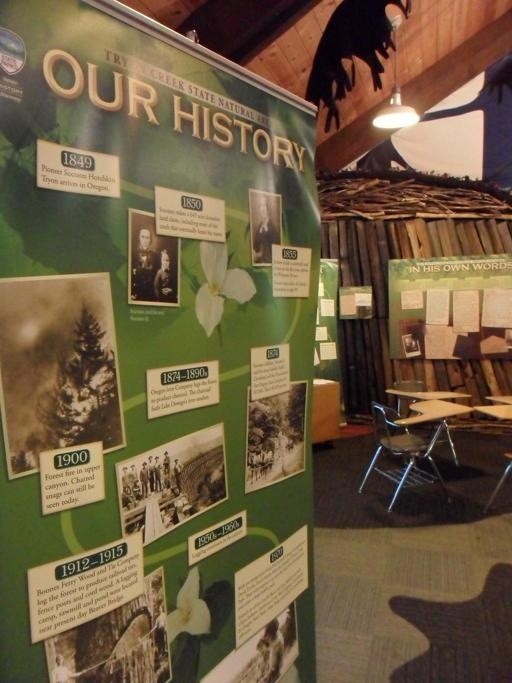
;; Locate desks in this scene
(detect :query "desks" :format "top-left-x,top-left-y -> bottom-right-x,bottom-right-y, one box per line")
474,404 -> 512,514
485,395 -> 512,405
385,388 -> 472,450
393,399 -> 474,472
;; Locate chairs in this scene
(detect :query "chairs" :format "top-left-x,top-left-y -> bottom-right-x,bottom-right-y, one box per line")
393,380 -> 451,442
356,401 -> 451,513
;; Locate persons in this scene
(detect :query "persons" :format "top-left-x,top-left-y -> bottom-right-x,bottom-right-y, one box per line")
254,620 -> 285,682
251,194 -> 280,264
120,452 -> 184,508
130,229 -> 176,301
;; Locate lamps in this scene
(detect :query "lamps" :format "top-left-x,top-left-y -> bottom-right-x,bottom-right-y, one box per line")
371,16 -> 421,131
